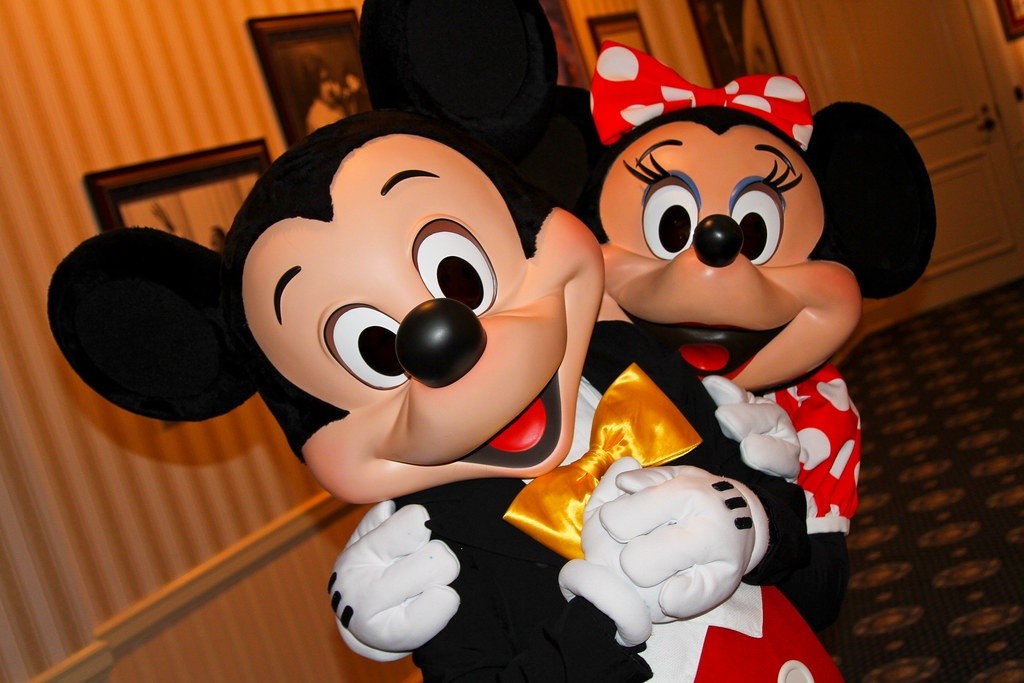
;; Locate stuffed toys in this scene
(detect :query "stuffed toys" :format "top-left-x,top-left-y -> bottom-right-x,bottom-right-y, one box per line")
47,0 -> 846,683
516,39 -> 937,683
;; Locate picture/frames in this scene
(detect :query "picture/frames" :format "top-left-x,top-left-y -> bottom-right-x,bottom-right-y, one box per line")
536,0 -> 591,96
244,8 -> 375,150
81,135 -> 272,258
587,11 -> 651,57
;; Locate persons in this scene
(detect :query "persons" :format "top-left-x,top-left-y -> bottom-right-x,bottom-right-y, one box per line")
287,54 -> 361,136
208,227 -> 226,252
